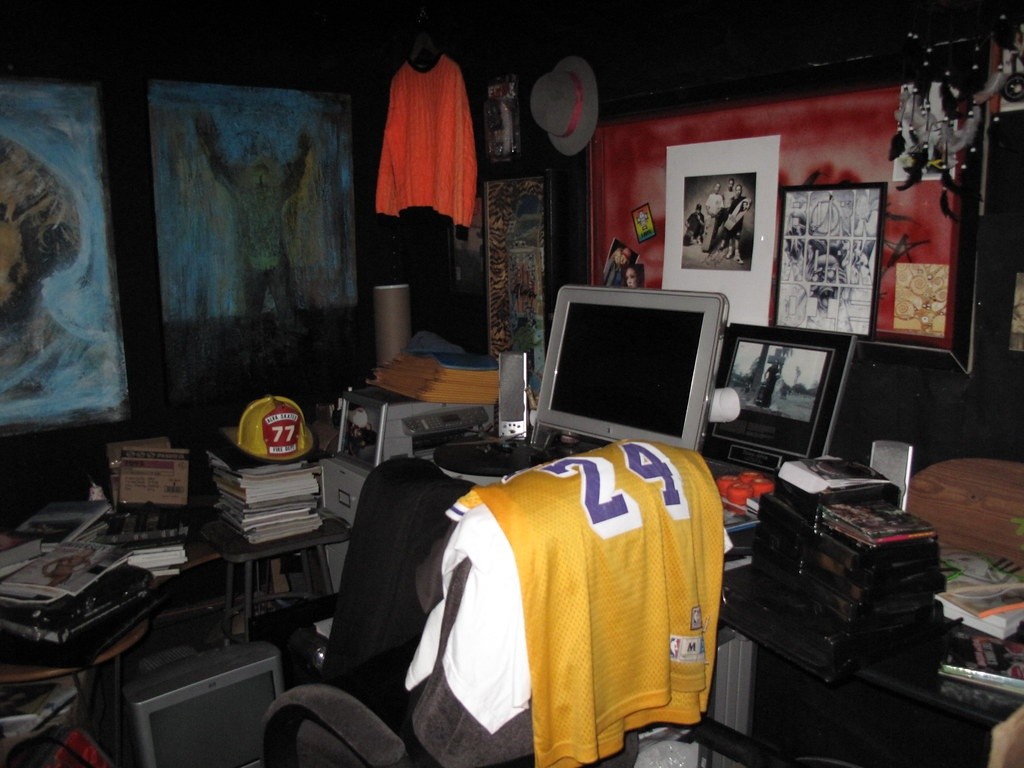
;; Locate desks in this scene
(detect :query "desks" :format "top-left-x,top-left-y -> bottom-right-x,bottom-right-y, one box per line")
0,542 -> 219,768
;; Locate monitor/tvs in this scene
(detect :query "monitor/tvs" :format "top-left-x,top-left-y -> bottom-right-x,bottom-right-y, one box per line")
536,284 -> 730,455
123,641 -> 286,768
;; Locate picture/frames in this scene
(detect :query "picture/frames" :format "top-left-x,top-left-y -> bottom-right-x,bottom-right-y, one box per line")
772,181 -> 889,341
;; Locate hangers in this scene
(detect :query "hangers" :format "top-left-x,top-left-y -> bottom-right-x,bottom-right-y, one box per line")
408,28 -> 442,63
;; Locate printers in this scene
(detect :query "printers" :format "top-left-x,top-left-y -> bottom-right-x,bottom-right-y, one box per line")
350,387 -> 495,465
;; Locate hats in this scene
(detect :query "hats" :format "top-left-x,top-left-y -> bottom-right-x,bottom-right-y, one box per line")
530,54 -> 598,155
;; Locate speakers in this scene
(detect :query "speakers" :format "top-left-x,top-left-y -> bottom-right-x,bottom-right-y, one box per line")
497,351 -> 528,441
870,441 -> 914,512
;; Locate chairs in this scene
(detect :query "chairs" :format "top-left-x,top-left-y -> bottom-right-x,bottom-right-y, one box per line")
258,443 -> 723,768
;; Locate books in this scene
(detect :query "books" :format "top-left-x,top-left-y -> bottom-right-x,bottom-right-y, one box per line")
935,544 -> 1024,694
0,450 -> 323,603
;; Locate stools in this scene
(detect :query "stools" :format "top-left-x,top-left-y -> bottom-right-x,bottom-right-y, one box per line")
202,513 -> 350,643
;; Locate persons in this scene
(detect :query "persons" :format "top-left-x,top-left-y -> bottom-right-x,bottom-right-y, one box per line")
604,178 -> 752,290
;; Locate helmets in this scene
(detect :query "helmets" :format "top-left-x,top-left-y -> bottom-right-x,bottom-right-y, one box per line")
236,395 -> 313,461
